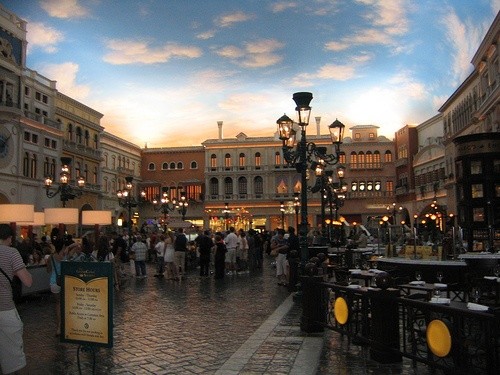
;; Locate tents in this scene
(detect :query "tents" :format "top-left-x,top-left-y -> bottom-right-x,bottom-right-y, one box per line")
164,218 -> 196,229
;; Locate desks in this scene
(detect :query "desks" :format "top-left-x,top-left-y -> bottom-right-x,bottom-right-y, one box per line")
397,282 -> 443,297
429,297 -> 489,312
349,269 -> 384,287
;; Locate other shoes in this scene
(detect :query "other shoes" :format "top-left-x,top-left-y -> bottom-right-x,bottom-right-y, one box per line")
137,276 -> 140,278
181,276 -> 185,281
284,282 -> 289,286
55,334 -> 60,338
176,277 -> 179,281
143,275 -> 148,277
278,282 -> 283,285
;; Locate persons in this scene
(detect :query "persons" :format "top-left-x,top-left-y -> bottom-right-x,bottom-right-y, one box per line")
47,239 -> 79,336
71,237 -> 101,262
307,225 -> 463,260
355,230 -> 368,248
14,226 -> 298,282
130,237 -> 148,279
0,224 -> 33,375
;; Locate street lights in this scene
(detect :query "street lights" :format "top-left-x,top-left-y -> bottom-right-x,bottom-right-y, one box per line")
44,164 -> 88,209
153,192 -> 178,235
176,195 -> 189,222
115,182 -> 147,249
386,203 -> 403,225
279,201 -> 287,231
273,91 -> 345,244
311,159 -> 348,242
293,195 -> 301,235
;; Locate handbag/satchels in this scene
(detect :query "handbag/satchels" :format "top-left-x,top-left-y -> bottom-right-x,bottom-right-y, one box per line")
270,250 -> 277,255
55,274 -> 62,287
11,275 -> 22,301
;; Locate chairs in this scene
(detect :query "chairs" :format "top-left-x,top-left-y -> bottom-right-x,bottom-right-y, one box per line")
446,284 -> 468,302
